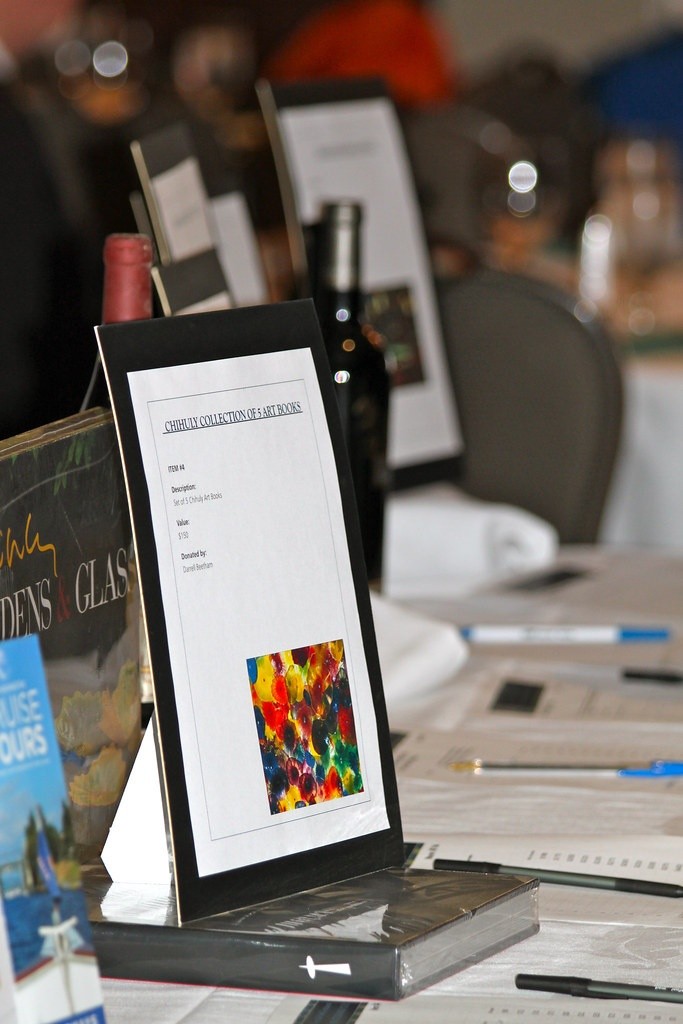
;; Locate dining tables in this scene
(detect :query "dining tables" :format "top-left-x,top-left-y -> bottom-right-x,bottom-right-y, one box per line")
97,546 -> 683,1024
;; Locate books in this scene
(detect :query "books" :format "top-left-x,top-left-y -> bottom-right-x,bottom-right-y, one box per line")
70,833 -> 541,1001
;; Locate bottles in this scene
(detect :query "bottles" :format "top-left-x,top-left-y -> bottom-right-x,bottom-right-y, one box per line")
302,200 -> 387,595
80,226 -> 159,418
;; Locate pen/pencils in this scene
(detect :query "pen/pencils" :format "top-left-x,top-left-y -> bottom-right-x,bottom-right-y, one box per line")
515,974 -> 682,1005
445,759 -> 682,780
459,618 -> 669,646
431,858 -> 683,899
